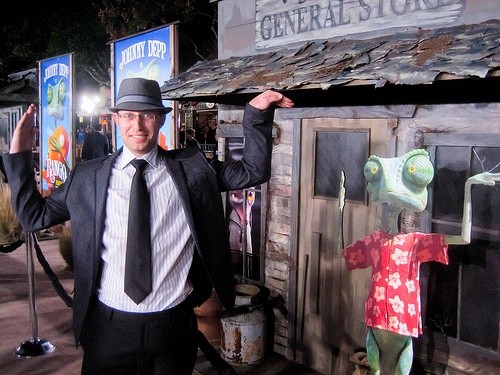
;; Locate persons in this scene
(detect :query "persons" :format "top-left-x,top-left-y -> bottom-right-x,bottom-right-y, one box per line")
77,123 -> 112,163
2,78 -> 295,375
179,121 -> 218,150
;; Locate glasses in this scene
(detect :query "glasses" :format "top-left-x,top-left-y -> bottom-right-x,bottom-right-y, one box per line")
117,113 -> 156,121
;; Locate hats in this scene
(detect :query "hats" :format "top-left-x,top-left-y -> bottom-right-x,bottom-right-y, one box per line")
108,78 -> 173,114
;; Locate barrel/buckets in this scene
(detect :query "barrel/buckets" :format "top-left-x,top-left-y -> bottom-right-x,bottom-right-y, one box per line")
235,283 -> 260,307
219,310 -> 266,366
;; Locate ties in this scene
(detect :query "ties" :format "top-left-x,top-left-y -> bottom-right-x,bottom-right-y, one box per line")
124,159 -> 152,304
